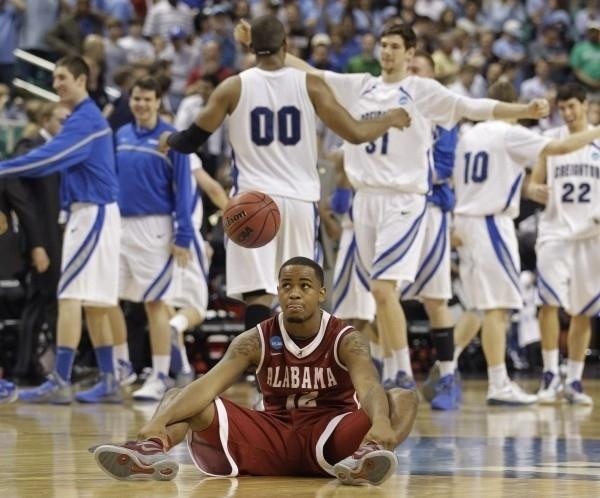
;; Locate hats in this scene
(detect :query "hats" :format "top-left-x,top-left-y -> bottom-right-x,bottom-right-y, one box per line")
212,6 -> 225,17
586,21 -> 600,31
310,33 -> 332,46
503,19 -> 526,38
168,27 -> 186,39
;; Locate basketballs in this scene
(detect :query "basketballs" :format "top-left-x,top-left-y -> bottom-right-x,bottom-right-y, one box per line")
222,192 -> 281,248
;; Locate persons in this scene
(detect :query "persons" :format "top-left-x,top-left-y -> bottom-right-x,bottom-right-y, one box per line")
0,0 -> 599,404
93,256 -> 417,484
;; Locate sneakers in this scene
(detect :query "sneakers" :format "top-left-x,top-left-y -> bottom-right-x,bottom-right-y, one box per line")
18,370 -> 72,404
75,327 -> 195,404
334,445 -> 398,486
508,347 -> 529,369
564,382 -> 592,405
486,381 -> 538,405
537,371 -> 564,403
384,363 -> 462,410
0,379 -> 19,404
94,441 -> 179,481
